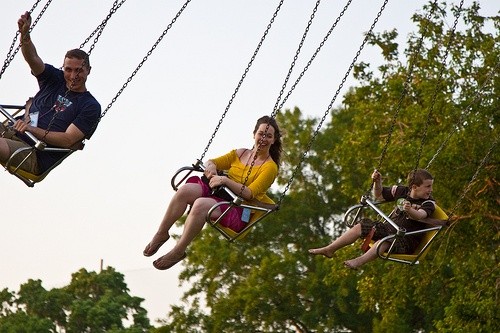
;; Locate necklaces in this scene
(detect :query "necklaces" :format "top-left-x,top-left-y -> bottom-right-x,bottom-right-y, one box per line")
240,151 -> 269,184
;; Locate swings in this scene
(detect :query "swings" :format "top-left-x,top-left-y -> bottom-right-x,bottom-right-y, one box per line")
343,0 -> 500,266
170,0 -> 390,244
0,0 -> 192,188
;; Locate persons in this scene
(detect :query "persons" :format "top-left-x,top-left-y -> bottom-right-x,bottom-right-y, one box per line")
0,10 -> 101,175
307,168 -> 436,267
143,115 -> 283,269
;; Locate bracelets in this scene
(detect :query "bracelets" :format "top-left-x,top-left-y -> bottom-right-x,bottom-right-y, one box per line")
22,39 -> 31,44
